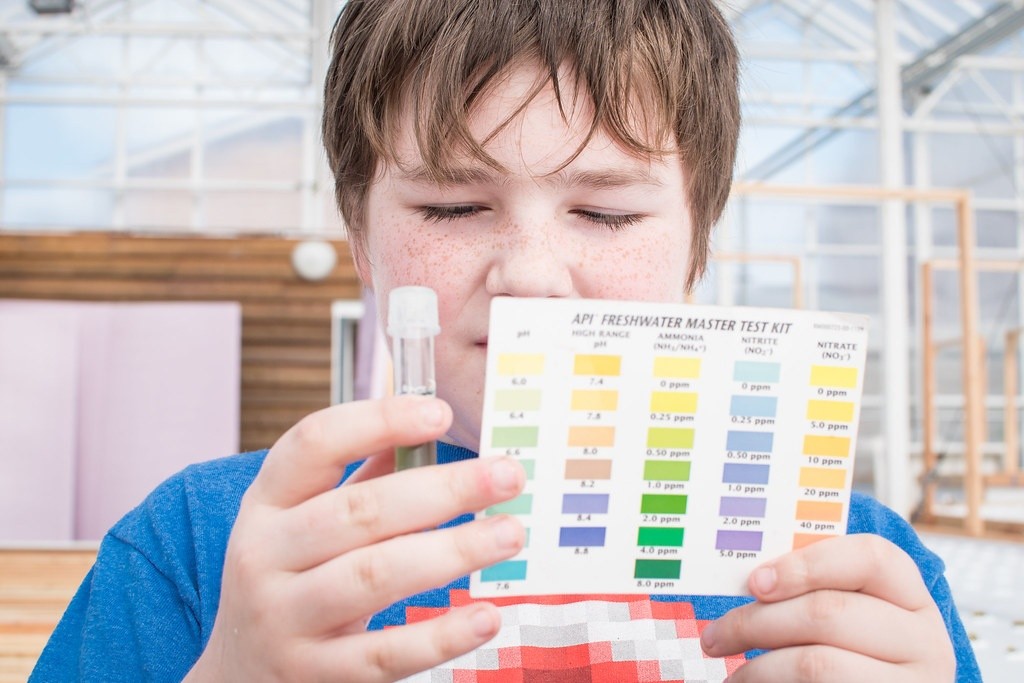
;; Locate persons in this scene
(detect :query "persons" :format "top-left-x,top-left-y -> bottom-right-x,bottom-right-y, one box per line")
27,0 -> 981,683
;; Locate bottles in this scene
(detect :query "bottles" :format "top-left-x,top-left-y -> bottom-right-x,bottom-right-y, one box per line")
384,286 -> 440,472
